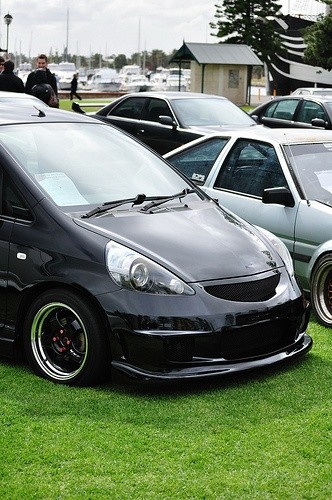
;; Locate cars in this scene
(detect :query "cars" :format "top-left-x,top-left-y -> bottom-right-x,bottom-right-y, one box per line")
249,88 -> 332,131
162,127 -> 332,328
0,89 -> 314,388
81,89 -> 269,155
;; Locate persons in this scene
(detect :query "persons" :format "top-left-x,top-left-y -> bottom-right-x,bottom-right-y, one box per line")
0,61 -> 25,93
0,56 -> 5,74
30,67 -> 59,108
70,74 -> 81,100
24,54 -> 57,94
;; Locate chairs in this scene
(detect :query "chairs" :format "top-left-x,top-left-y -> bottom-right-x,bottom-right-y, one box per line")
304,107 -> 319,123
151,107 -> 167,122
232,166 -> 276,196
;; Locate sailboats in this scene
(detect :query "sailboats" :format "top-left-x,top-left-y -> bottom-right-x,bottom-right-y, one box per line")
17,8 -> 188,95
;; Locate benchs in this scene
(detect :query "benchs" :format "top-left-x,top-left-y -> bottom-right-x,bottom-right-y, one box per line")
273,111 -> 325,119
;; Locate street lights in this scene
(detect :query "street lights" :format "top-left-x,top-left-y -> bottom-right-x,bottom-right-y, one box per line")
3,10 -> 13,61
